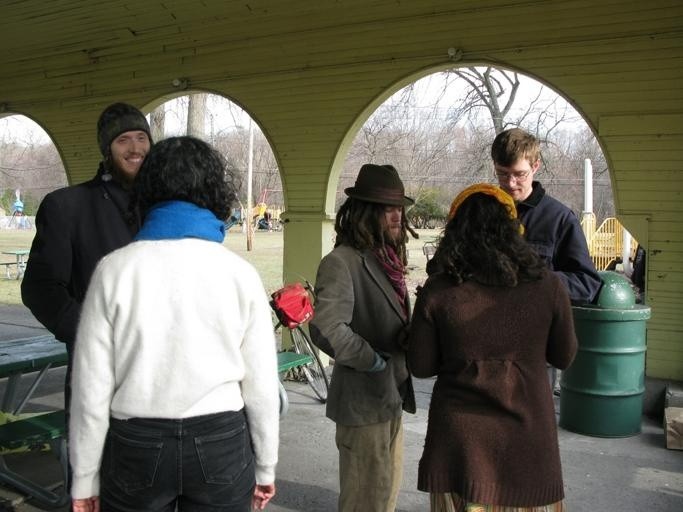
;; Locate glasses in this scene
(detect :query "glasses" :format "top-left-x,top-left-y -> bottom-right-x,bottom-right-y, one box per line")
493,170 -> 531,182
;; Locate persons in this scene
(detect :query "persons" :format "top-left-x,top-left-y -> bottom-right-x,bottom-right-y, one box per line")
65,136 -> 278,512
261,208 -> 273,231
16,99 -> 153,494
309,165 -> 418,512
402,182 -> 580,512
489,128 -> 604,309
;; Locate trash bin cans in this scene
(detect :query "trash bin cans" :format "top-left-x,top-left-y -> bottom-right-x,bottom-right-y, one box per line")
560,269 -> 651,438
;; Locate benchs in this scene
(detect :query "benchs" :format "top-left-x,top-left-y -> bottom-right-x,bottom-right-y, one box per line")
1,408 -> 71,508
276,349 -> 312,420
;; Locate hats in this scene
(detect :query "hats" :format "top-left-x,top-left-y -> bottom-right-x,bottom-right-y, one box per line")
344,165 -> 415,206
97,103 -> 154,156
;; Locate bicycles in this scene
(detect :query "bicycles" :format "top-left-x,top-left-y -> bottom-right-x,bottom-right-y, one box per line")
268,283 -> 330,404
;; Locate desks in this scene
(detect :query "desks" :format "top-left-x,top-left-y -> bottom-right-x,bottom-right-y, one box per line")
1,334 -> 69,421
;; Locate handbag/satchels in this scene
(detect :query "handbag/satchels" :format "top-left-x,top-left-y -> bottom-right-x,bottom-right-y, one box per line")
270,284 -> 315,329
